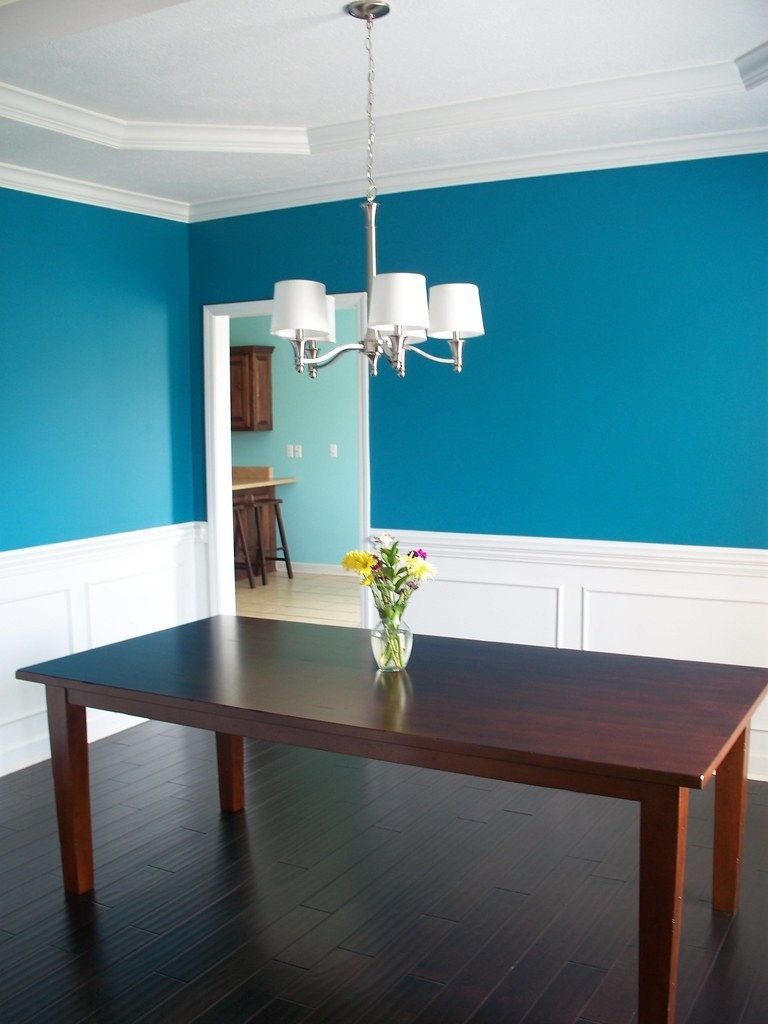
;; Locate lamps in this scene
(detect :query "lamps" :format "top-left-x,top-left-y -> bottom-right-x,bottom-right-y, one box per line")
271,0 -> 487,378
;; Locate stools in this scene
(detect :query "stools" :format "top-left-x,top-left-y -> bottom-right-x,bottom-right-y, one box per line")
234,498 -> 292,589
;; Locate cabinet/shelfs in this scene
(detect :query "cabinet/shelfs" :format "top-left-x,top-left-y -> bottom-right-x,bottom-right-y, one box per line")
232,465 -> 295,580
230,345 -> 276,431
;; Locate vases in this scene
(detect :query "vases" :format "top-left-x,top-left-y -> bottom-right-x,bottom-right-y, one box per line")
371,616 -> 413,671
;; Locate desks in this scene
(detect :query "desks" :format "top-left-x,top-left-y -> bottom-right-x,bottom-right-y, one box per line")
16,615 -> 767,1024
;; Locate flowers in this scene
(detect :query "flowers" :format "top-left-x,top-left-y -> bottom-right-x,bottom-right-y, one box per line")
342,535 -> 436,671
